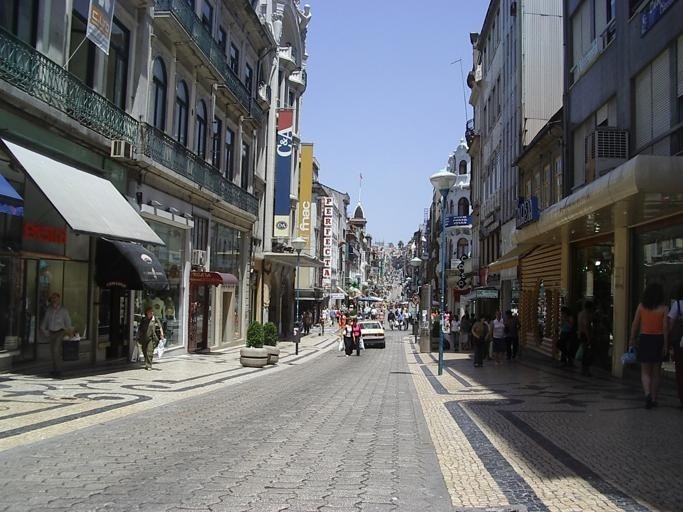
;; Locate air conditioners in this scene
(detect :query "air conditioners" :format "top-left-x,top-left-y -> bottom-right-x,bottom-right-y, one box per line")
110,139 -> 133,161
192,249 -> 207,267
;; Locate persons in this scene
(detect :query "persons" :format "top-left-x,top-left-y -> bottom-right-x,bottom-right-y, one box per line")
629,283 -> 683,409
136,308 -> 165,370
431,308 -> 522,367
560,300 -> 597,377
40,293 -> 75,379
298,303 -> 414,357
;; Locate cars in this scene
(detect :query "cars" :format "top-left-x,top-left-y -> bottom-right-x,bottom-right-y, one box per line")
355,320 -> 387,350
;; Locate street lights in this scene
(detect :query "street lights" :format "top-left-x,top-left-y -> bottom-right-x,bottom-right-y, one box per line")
429,169 -> 458,377
290,236 -> 306,342
355,273 -> 362,289
410,255 -> 423,336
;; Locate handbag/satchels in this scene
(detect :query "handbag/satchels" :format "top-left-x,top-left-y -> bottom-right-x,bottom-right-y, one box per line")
338,338 -> 345,351
623,345 -> 637,366
359,337 -> 365,350
673,300 -> 683,346
128,341 -> 146,362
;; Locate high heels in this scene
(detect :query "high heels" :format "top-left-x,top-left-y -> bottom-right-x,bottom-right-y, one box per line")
644,394 -> 656,410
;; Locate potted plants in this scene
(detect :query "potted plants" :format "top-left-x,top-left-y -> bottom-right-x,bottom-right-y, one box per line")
239,320 -> 269,368
264,321 -> 280,364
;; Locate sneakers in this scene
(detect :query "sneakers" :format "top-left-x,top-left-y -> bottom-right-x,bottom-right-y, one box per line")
145,365 -> 153,370
474,355 -> 519,368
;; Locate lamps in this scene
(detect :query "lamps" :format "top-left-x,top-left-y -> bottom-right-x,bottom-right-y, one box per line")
146,199 -> 192,220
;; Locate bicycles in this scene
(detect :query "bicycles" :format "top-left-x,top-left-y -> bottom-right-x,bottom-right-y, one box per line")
395,319 -> 403,331
